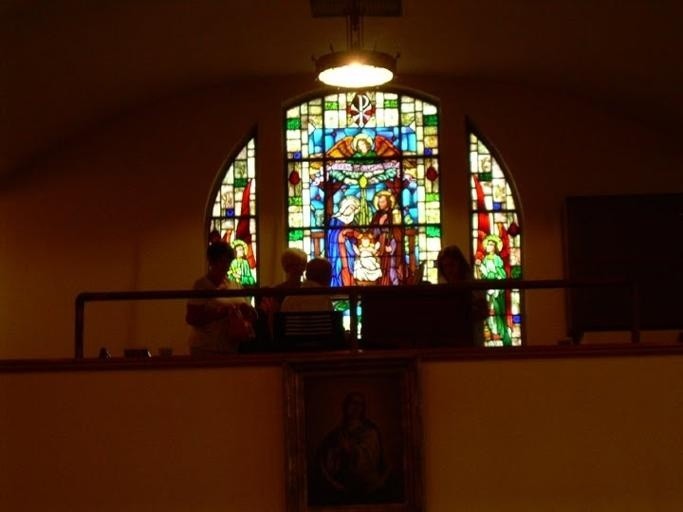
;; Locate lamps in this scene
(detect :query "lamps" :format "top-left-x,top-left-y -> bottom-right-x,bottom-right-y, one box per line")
308,0 -> 399,91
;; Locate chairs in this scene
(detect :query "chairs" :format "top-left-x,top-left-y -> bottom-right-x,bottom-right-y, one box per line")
268,311 -> 344,351
359,285 -> 471,347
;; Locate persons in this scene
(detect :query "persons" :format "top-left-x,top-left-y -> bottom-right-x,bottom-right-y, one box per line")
475,236 -> 512,348
365,191 -> 408,286
438,245 -> 488,346
317,391 -> 385,504
185,241 -> 258,357
227,241 -> 255,305
326,197 -> 364,287
351,138 -> 380,165
275,259 -> 333,335
259,248 -> 307,333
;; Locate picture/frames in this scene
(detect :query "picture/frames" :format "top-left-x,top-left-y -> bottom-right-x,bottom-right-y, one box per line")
282,357 -> 423,510
